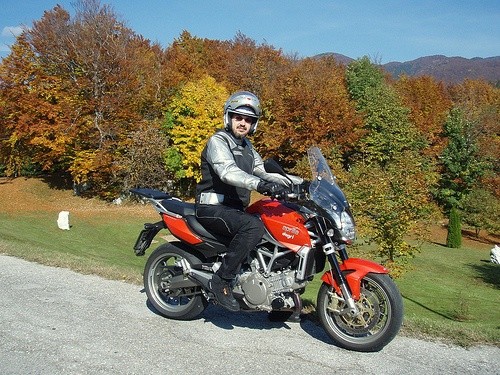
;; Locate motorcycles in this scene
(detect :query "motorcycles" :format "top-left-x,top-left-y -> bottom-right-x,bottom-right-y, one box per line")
127,146 -> 404,354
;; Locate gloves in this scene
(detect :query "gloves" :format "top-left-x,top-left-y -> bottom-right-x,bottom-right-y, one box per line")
301,180 -> 312,194
258,180 -> 286,199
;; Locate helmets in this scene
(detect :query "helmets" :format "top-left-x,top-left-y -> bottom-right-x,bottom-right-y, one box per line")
224,91 -> 262,135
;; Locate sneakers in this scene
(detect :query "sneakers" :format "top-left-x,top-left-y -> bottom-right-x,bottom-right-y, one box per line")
207,274 -> 241,313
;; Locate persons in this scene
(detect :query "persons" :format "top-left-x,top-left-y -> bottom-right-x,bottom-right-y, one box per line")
194,91 -> 312,313
